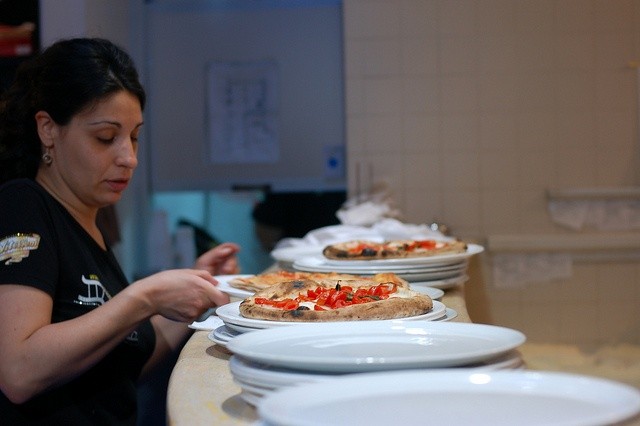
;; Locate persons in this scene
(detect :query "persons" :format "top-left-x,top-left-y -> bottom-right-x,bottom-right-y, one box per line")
251,201 -> 289,252
2,36 -> 240,426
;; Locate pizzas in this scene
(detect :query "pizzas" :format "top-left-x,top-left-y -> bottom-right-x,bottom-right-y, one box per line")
324,241 -> 467,260
238,282 -> 433,319
227,273 -> 410,295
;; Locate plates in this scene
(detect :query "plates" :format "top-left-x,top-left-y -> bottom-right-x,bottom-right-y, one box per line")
227,319 -> 526,412
241,368 -> 639,426
208,274 -> 445,299
268,234 -> 485,290
207,295 -> 458,348
